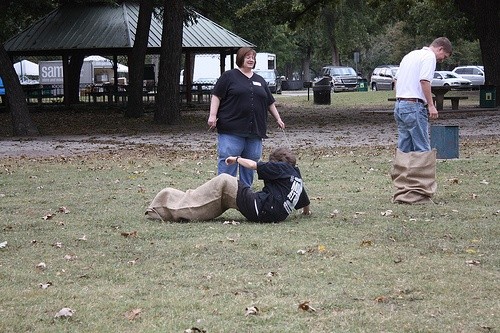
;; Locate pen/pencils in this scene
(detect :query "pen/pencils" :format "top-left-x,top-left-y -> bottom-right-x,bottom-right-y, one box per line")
207,118 -> 220,133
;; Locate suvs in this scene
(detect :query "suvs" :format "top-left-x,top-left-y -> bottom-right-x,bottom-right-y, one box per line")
451,65 -> 485,90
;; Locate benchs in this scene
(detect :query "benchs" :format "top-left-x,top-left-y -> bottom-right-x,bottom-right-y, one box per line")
85,90 -> 213,97
387,97 -> 468,102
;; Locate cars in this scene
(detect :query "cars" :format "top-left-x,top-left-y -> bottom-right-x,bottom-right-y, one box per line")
431,71 -> 472,91
318,66 -> 359,93
370,64 -> 400,92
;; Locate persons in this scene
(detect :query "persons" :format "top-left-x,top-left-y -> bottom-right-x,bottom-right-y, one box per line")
392,38 -> 453,205
146,147 -> 317,221
207,47 -> 285,189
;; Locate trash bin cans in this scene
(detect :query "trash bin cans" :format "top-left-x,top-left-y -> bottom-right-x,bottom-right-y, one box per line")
313,85 -> 331,105
43,84 -> 52,96
479,84 -> 496,107
358,78 -> 368,92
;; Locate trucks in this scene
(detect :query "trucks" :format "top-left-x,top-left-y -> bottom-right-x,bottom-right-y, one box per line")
179,51 -> 278,97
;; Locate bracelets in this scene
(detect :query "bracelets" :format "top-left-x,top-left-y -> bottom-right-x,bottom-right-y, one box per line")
236,156 -> 240,163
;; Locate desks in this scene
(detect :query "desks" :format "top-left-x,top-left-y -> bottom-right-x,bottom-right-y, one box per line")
430,85 -> 460,111
192,83 -> 213,104
143,85 -> 157,102
91,85 -> 105,102
